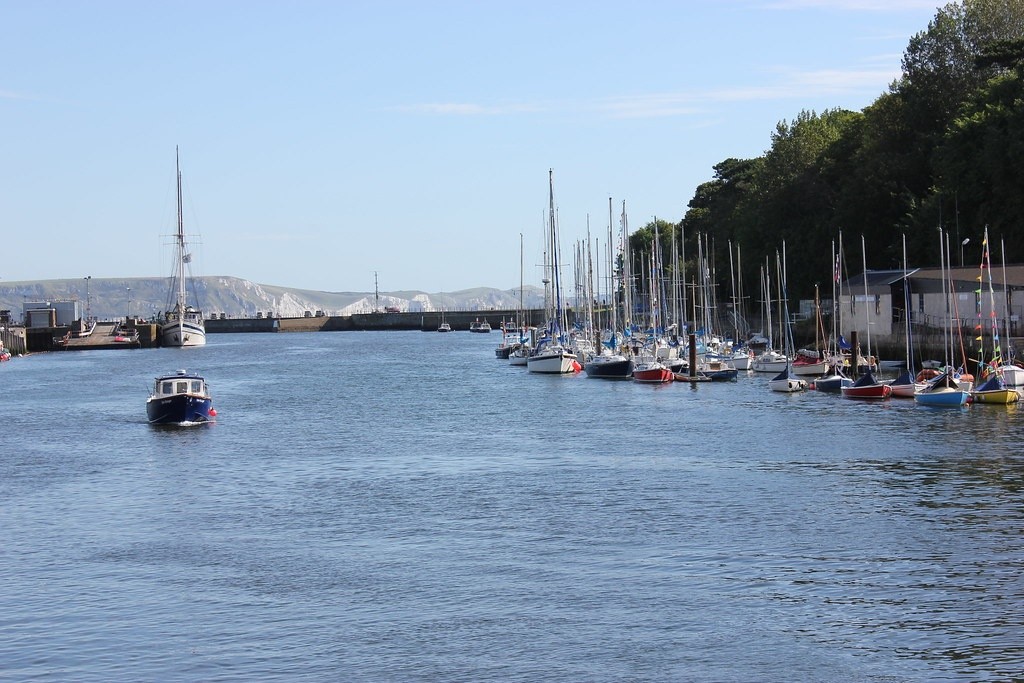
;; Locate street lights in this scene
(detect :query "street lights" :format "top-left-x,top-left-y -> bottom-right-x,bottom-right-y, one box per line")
127,288 -> 130,317
84,276 -> 91,320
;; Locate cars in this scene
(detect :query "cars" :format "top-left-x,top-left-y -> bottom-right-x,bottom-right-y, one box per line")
388,306 -> 400,312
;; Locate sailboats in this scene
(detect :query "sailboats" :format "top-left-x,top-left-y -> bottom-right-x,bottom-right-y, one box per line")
159,145 -> 207,346
494,168 -> 1024,406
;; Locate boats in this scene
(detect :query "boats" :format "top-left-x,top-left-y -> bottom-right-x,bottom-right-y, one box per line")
438,292 -> 451,331
145,368 -> 213,426
470,318 -> 491,333
135,324 -> 161,348
